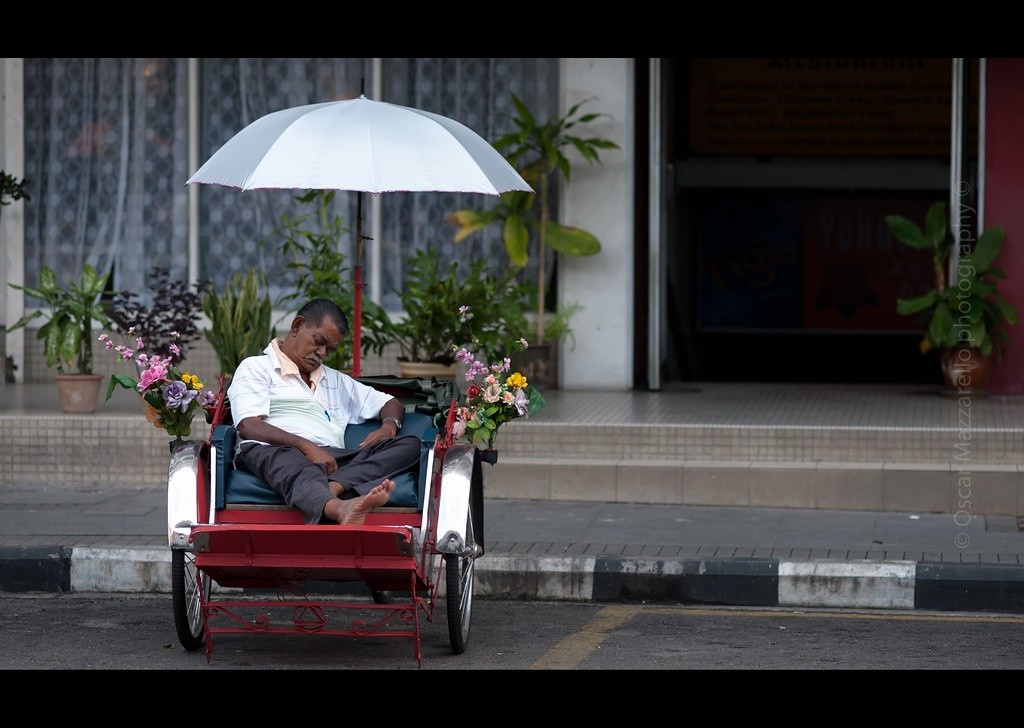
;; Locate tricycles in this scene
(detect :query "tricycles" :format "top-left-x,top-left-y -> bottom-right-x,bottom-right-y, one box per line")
168,382 -> 486,670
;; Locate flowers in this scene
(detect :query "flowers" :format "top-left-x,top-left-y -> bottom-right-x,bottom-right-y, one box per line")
448,303 -> 545,450
96,323 -> 213,442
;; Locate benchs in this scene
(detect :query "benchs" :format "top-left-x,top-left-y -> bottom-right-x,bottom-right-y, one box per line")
217,411 -> 437,515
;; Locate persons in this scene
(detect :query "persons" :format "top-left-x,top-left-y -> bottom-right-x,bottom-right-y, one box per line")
227,298 -> 421,526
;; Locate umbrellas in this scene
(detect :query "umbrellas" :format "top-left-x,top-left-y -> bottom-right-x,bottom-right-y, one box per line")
184,95 -> 537,377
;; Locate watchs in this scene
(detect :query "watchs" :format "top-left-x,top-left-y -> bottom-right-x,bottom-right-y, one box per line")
383,417 -> 401,428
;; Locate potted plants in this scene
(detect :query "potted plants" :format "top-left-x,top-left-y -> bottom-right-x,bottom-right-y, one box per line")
6,263 -> 121,414
102,267 -> 208,426
442,87 -> 621,391
201,272 -> 282,391
259,186 -> 397,376
884,202 -> 1020,390
395,241 -> 485,377
